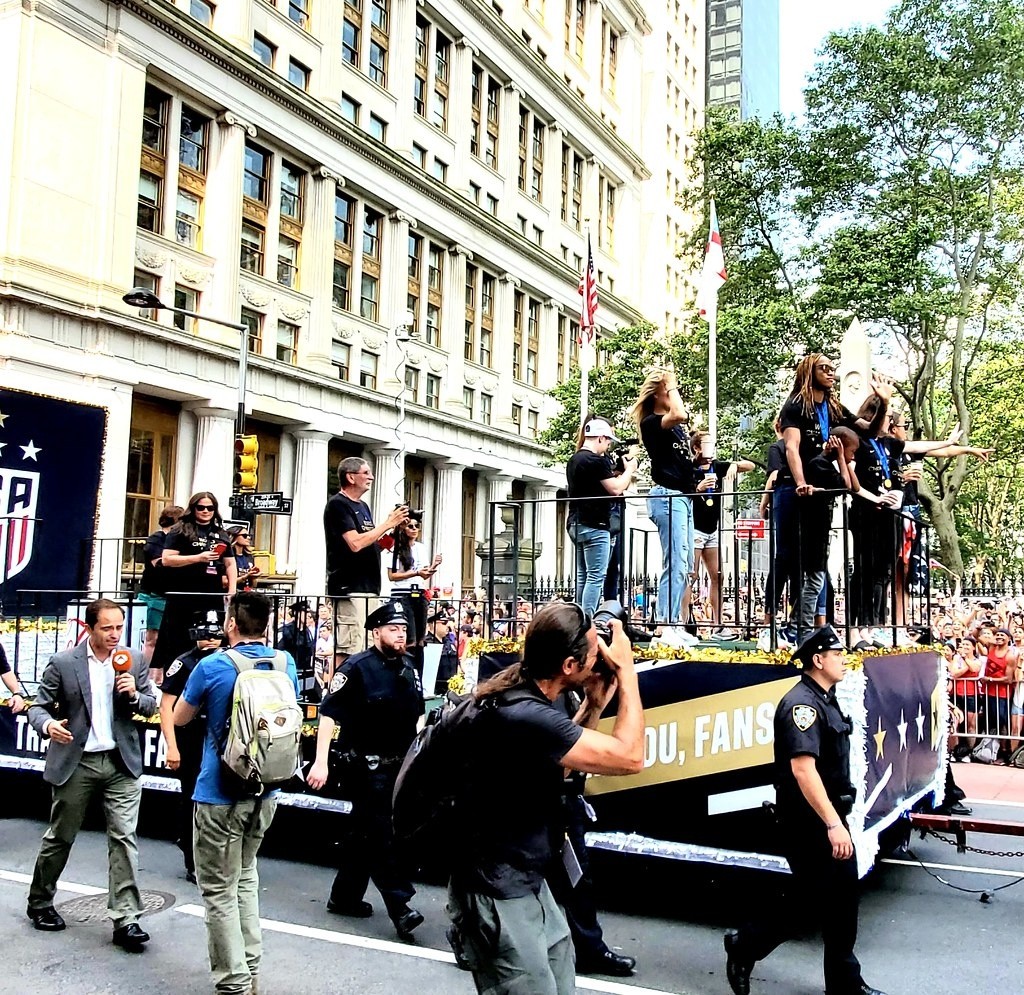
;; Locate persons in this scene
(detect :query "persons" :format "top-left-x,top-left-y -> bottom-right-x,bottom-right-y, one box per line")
425,595 -> 1023,767
724,624 -> 886,994
681,429 -> 756,640
26,601 -> 157,945
263,600 -> 335,701
226,526 -> 260,592
387,509 -> 443,681
447,605 -> 646,995
759,353 -> 996,651
306,602 -> 426,935
632,363 -> 704,640
566,413 -> 653,641
158,611 -> 224,885
323,457 -> 409,669
551,688 -> 636,974
138,505 -> 185,684
149,491 -> 237,685
0,643 -> 25,713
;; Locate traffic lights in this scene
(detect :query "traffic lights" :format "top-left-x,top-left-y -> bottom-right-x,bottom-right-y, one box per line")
232,434 -> 259,490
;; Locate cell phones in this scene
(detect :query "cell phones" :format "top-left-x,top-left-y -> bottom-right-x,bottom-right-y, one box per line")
211,544 -> 227,559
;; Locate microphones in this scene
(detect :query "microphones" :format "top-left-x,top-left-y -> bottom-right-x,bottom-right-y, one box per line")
616,438 -> 639,445
112,651 -> 131,697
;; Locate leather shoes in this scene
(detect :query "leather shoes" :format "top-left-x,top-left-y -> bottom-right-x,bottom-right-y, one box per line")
27,906 -> 150,947
725,930 -> 889,995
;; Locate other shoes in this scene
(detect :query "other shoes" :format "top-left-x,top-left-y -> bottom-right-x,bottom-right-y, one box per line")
575,948 -> 635,972
870,629 -> 890,648
326,898 -> 426,937
756,628 -> 790,652
651,626 -> 701,652
897,629 -> 917,648
962,756 -> 970,762
779,626 -> 798,649
950,755 -> 956,762
445,926 -> 472,971
711,626 -> 740,642
953,801 -> 974,814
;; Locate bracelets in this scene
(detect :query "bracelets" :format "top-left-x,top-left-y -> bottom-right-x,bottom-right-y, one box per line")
828,819 -> 843,831
665,387 -> 678,395
731,462 -> 741,469
12,691 -> 25,700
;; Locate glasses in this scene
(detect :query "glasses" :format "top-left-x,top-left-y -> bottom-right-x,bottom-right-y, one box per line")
347,470 -> 371,475
238,533 -> 251,539
886,413 -> 894,422
408,523 -> 420,528
897,422 -> 910,430
436,621 -> 448,625
195,504 -> 214,511
561,601 -> 592,649
816,364 -> 836,373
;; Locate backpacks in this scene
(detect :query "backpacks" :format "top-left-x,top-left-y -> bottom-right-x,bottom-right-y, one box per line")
211,647 -> 303,788
1010,745 -> 1024,768
391,686 -> 555,848
973,729 -> 1000,763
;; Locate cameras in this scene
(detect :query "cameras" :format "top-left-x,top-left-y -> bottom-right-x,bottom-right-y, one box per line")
395,504 -> 424,523
592,600 -> 628,673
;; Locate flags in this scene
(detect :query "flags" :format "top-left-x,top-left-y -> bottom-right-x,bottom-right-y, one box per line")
694,198 -> 729,325
576,230 -> 602,349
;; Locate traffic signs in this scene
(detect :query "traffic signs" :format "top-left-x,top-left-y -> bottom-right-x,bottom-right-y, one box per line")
253,497 -> 294,516
242,492 -> 286,510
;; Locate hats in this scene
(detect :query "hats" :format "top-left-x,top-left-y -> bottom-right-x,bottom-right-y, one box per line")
189,610 -> 226,640
996,628 -> 1011,636
427,610 -> 451,623
292,600 -> 311,611
584,420 -> 621,443
790,621 -> 845,666
365,601 -> 411,630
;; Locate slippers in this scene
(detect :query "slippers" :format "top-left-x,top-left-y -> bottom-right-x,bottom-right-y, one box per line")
852,640 -> 883,656
